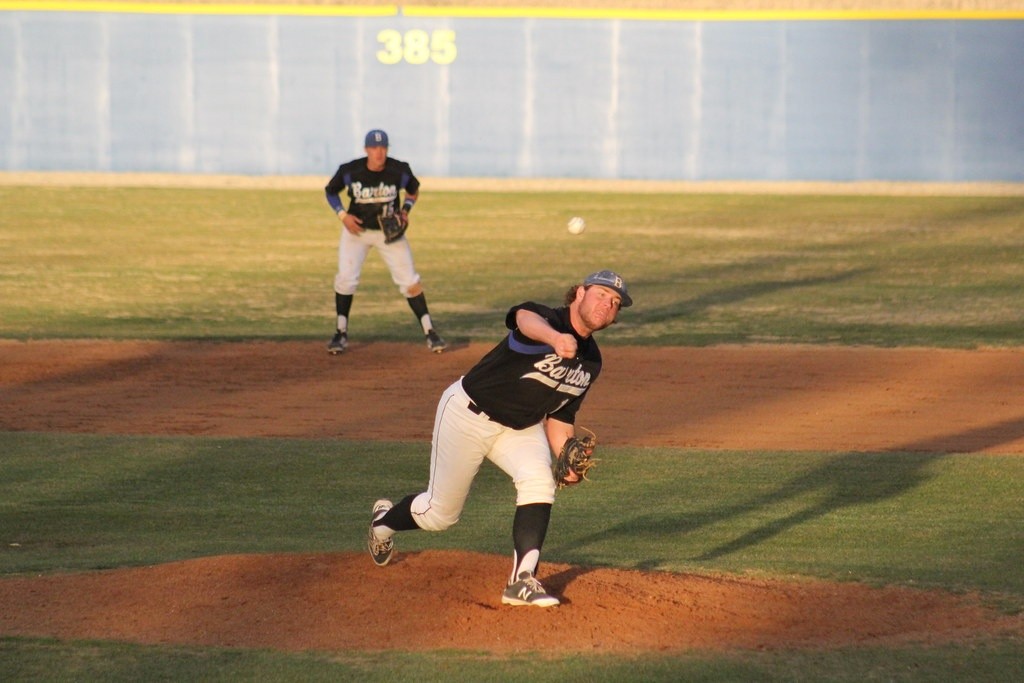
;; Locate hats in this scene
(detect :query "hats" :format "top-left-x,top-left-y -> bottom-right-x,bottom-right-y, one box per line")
365,130 -> 388,146
582,270 -> 633,307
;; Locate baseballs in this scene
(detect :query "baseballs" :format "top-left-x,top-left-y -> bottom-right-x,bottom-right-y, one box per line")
567,216 -> 585,235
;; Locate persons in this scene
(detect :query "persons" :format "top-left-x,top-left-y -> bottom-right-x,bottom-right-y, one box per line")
326,129 -> 449,352
368,272 -> 632,608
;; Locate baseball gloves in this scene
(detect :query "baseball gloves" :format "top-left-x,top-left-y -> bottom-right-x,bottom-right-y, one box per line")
377,211 -> 408,245
553,436 -> 596,488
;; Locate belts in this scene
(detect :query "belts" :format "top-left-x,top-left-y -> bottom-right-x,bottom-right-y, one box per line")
468,401 -> 498,422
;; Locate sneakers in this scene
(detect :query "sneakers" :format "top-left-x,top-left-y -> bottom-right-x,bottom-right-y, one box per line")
367,499 -> 397,566
501,570 -> 560,607
327,329 -> 350,352
425,329 -> 448,352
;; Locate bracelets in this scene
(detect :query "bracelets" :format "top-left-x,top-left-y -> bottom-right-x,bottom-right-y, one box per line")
403,198 -> 415,212
